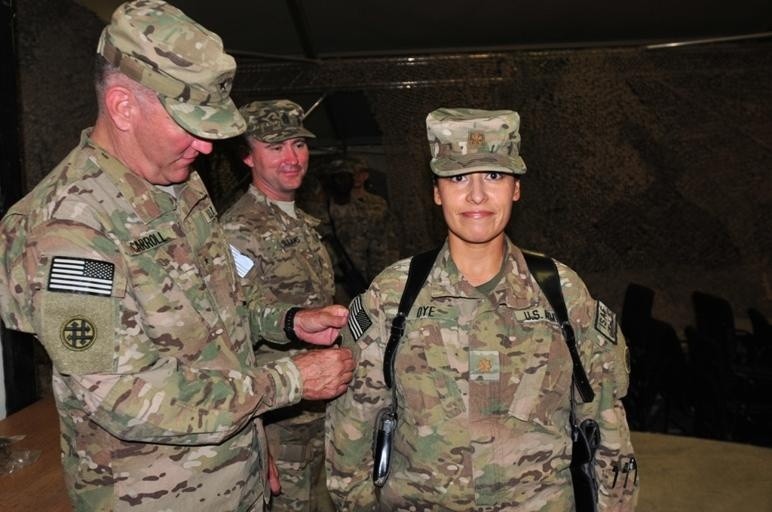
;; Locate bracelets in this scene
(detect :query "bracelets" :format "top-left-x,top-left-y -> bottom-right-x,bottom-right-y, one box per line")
285,307 -> 305,343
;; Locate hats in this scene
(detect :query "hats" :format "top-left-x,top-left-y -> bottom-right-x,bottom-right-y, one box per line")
97,0 -> 248,142
237,96 -> 318,144
423,105 -> 528,176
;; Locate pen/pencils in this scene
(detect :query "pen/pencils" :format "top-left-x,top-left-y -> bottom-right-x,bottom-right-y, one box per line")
612,458 -> 637,488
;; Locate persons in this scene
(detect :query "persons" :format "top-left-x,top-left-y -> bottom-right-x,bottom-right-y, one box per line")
0,0 -> 356,512
219,99 -> 337,512
324,107 -> 640,512
